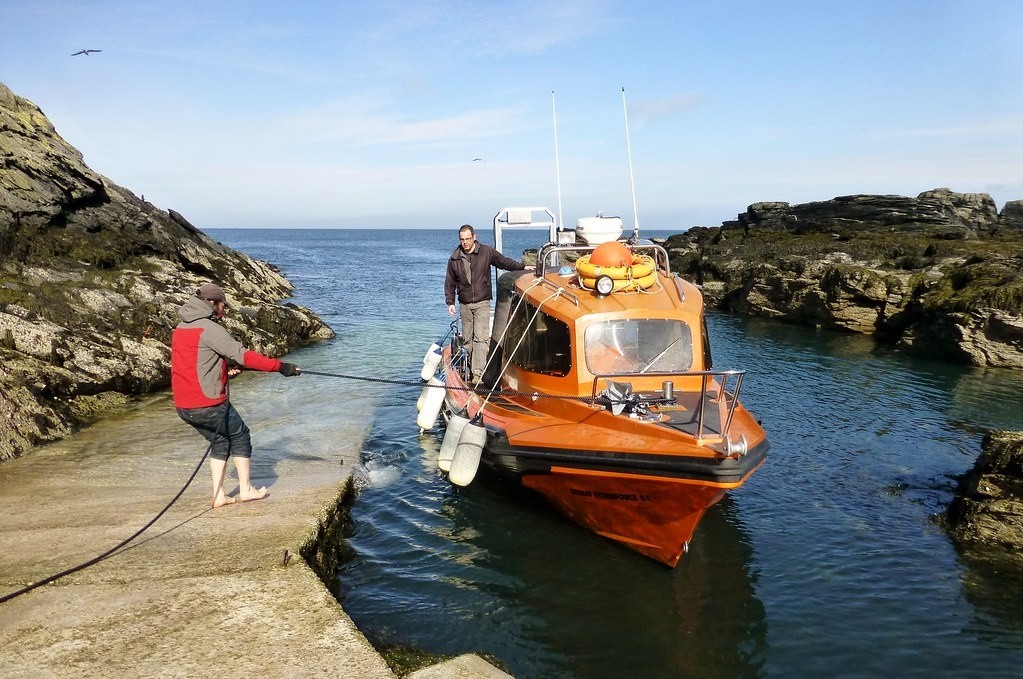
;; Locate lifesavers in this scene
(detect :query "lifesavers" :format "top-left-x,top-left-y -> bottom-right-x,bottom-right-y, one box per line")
583,271 -> 656,290
577,253 -> 654,279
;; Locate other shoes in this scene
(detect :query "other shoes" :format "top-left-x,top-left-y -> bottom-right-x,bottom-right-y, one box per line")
472,377 -> 482,384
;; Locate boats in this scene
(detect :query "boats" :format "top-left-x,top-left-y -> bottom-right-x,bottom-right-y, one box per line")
440,216 -> 774,574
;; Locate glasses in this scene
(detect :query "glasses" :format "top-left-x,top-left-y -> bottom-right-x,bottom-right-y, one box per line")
460,237 -> 473,243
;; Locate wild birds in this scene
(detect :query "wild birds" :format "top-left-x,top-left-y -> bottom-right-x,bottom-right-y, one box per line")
473,159 -> 486,162
72,49 -> 102,56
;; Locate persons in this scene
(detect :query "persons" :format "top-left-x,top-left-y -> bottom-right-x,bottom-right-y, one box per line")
170,283 -> 300,507
443,224 -> 536,385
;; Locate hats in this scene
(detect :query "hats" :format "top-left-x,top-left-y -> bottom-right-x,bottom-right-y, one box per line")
196,283 -> 230,306
